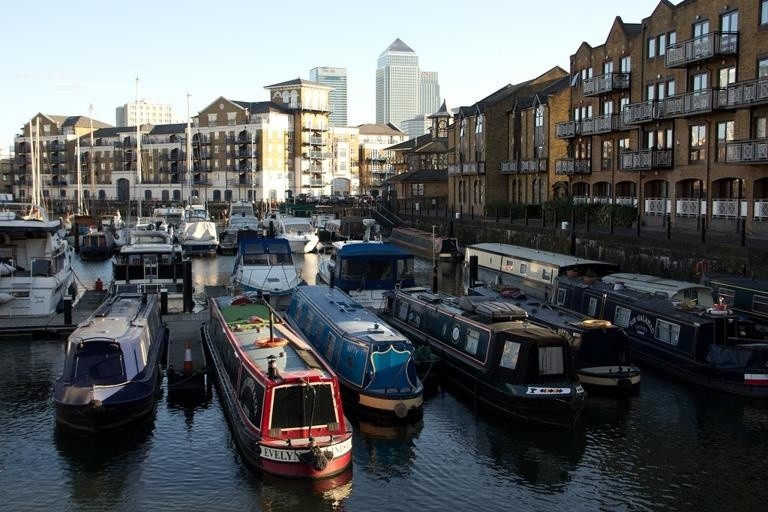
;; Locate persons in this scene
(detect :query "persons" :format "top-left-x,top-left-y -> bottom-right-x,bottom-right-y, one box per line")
694,258 -> 710,283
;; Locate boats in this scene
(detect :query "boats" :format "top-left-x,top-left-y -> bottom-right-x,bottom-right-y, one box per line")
200,290 -> 354,481
285,284 -> 425,419
49,292 -> 169,435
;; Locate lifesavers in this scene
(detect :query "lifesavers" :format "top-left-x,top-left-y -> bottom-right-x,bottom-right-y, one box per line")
706,307 -> 733,316
255,337 -> 289,348
583,319 -> 612,329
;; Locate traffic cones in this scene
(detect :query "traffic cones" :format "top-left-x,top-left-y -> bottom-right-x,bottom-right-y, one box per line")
183,339 -> 194,372
